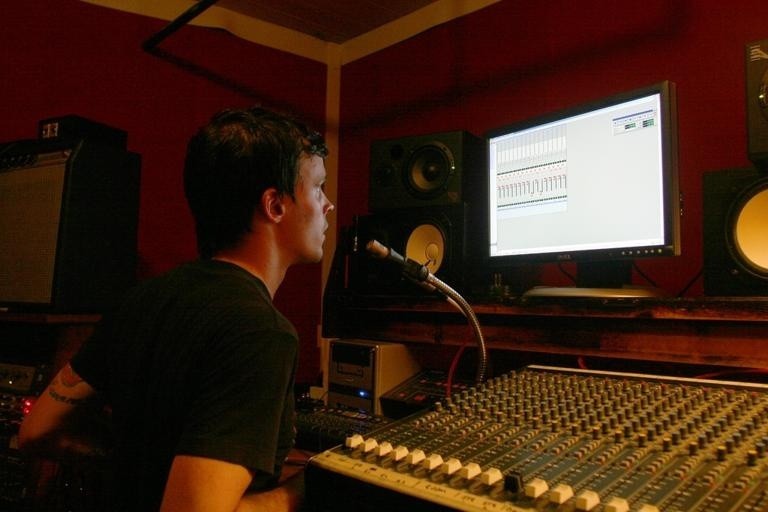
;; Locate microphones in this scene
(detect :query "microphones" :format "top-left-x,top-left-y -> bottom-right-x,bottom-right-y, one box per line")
366,238 -> 429,280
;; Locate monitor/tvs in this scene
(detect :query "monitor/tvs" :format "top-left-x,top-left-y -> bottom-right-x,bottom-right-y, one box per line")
483,81 -> 682,299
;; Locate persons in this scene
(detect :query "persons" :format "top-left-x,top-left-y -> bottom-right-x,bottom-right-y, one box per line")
15,102 -> 340,512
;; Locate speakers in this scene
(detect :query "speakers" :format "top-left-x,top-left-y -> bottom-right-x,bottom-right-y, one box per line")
369,129 -> 484,214
745,40 -> 768,163
0,139 -> 140,314
703,167 -> 768,296
350,215 -> 485,299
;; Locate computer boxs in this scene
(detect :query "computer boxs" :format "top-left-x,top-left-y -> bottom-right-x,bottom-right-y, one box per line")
327,338 -> 448,415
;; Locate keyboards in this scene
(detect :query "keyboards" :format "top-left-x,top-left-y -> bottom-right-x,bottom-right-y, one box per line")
296,405 -> 398,444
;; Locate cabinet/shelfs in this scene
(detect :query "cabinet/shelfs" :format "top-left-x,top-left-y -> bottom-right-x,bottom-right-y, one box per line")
275,297 -> 768,512
0,311 -> 114,512
